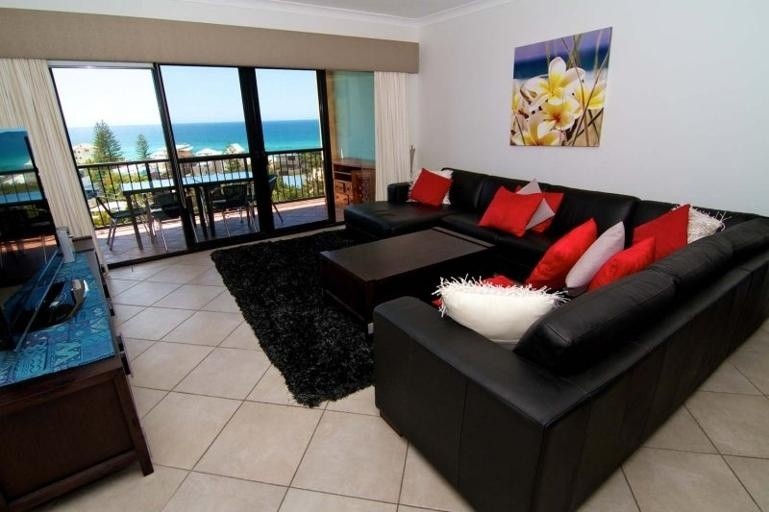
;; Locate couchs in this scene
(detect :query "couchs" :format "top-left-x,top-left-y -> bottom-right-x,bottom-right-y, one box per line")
385,166 -> 763,279
372,215 -> 768,512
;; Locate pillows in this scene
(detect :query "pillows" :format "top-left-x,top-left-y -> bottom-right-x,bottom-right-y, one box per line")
633,204 -> 691,261
515,178 -> 555,229
566,222 -> 625,288
523,217 -> 597,290
672,202 -> 723,245
589,235 -> 656,292
408,169 -> 454,207
516,185 -> 563,233
432,273 -> 572,350
479,187 -> 545,237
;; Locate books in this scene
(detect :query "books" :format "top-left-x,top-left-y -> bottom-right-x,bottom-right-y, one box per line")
56,226 -> 77,264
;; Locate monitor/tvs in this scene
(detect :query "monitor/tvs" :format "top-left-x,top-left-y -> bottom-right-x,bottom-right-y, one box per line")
0,129 -> 89,353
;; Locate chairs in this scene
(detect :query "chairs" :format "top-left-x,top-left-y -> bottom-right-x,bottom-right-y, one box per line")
210,184 -> 259,238
151,191 -> 199,253
241,175 -> 284,227
197,184 -> 227,228
95,195 -> 154,251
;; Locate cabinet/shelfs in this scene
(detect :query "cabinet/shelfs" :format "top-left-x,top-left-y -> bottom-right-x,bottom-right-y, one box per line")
333,158 -> 376,207
0,236 -> 155,512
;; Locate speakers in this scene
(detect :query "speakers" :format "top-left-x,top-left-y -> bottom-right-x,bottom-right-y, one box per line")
56,227 -> 77,263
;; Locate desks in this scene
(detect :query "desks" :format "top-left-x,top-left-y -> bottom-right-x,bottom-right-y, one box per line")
316,226 -> 496,346
120,170 -> 254,250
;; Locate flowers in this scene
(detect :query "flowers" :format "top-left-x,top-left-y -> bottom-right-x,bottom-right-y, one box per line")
510,56 -> 606,147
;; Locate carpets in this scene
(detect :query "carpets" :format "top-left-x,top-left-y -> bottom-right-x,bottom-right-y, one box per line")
210,223 -> 375,411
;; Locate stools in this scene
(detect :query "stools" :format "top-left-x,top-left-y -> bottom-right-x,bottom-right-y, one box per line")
341,200 -> 454,239
